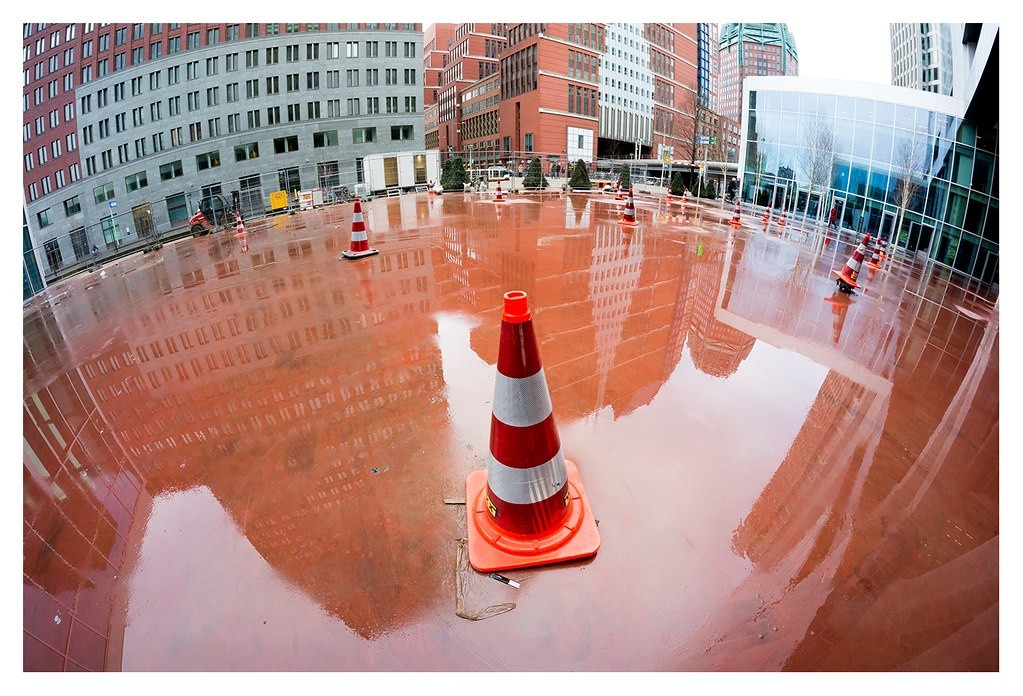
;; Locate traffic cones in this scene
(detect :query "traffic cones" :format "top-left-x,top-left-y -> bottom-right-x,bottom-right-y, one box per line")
761,221 -> 769,234
680,188 -> 689,203
879,236 -> 887,259
341,198 -> 379,259
832,232 -> 871,289
494,201 -> 504,223
428,179 -> 437,195
825,297 -> 858,346
615,181 -> 624,200
492,178 -> 506,202
867,237 -> 881,268
617,188 -> 640,226
664,186 -> 674,201
466,290 -> 601,573
728,199 -> 742,226
776,207 -> 787,226
777,225 -> 785,238
618,222 -> 637,258
761,203 -> 770,222
235,211 -> 248,237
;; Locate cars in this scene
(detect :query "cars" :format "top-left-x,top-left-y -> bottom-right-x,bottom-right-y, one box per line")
188,194 -> 240,237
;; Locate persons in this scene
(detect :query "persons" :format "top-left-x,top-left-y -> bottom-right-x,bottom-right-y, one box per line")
846,213 -> 854,230
516,160 -> 561,179
728,177 -> 737,203
827,205 -> 838,229
91,244 -> 98,254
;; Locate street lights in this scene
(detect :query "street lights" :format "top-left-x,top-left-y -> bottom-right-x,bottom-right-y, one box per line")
452,121 -> 466,157
